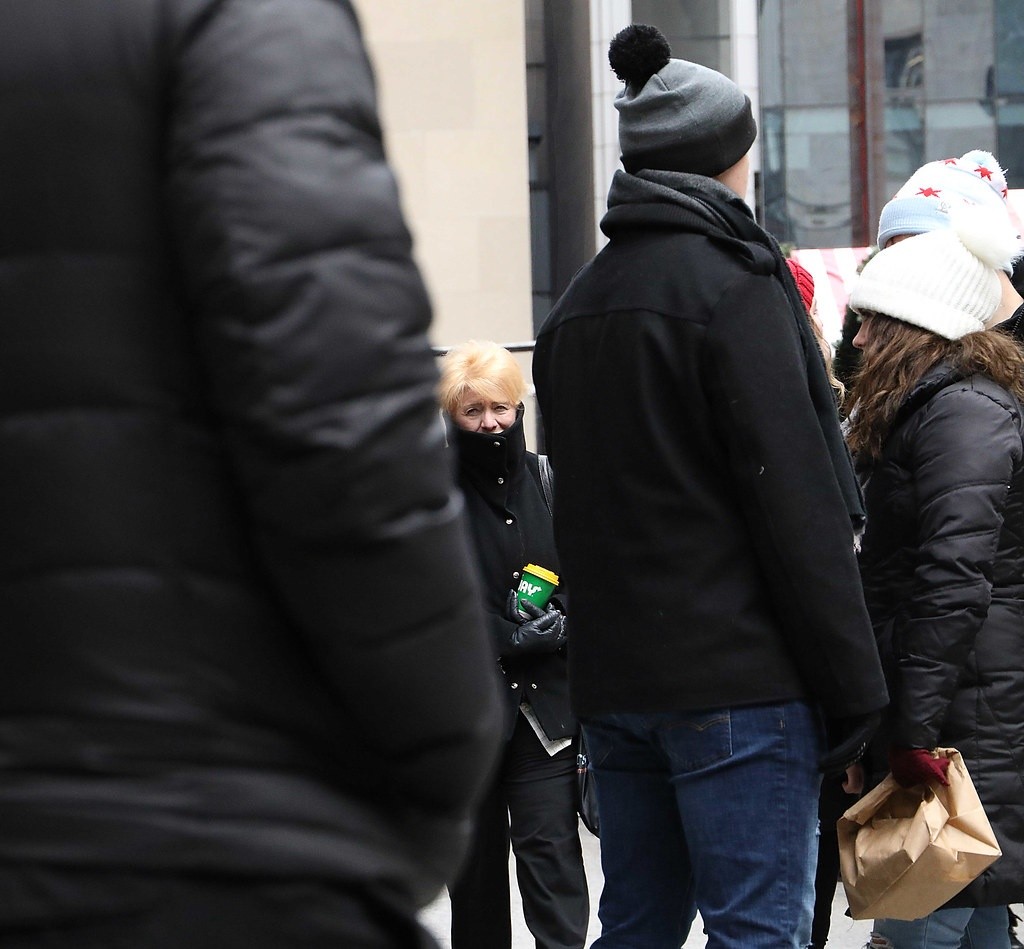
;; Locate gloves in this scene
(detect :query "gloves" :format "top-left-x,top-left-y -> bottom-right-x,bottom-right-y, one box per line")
505,603 -> 567,659
888,745 -> 951,789
503,588 -> 554,624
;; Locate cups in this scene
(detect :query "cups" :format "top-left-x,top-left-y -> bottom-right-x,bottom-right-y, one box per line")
512,562 -> 561,620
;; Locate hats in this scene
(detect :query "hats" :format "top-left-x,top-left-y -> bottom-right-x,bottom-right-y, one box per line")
777,258 -> 814,316
847,209 -> 1024,343
607,22 -> 758,178
877,150 -> 1015,277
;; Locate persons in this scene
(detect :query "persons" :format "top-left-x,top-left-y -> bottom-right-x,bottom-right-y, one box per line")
530,19 -> 888,949
828,215 -> 1024,949
776,250 -> 827,363
422,336 -> 598,949
0,0 -> 506,949
833,141 -> 1024,949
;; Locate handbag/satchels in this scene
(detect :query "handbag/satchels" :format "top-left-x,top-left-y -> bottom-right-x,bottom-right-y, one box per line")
575,732 -> 603,838
